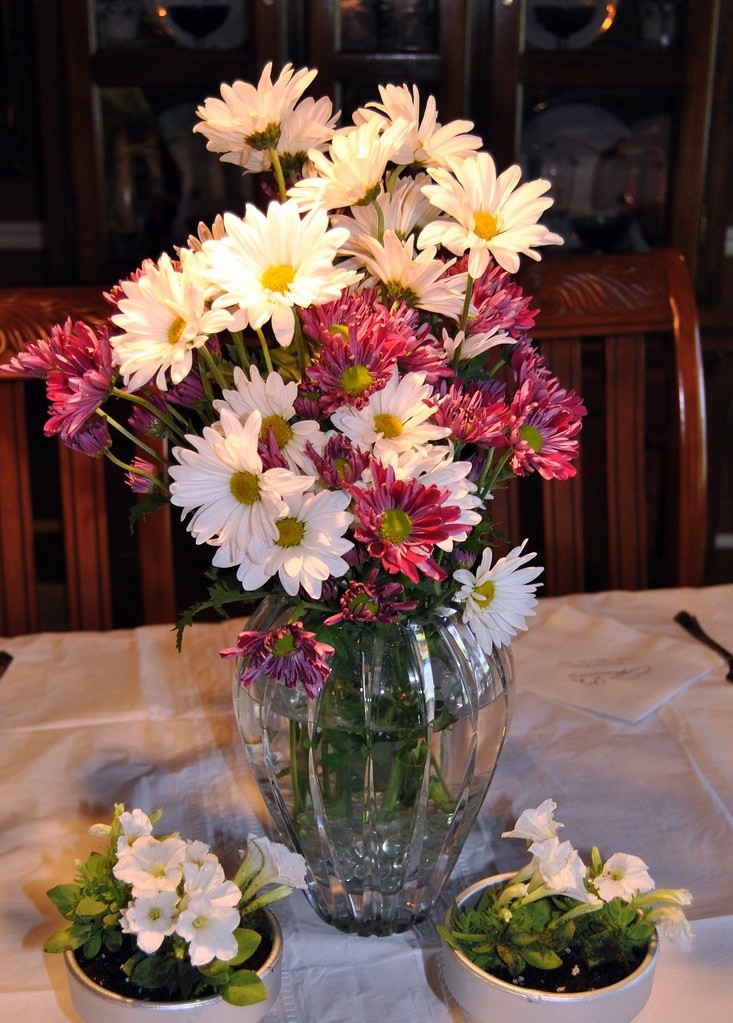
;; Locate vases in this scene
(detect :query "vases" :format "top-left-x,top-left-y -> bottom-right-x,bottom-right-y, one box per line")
64,907 -> 283,1023
231,589 -> 515,939
442,872 -> 658,1023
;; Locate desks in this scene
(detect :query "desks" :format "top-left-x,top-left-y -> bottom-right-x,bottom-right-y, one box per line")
0,584 -> 733,1021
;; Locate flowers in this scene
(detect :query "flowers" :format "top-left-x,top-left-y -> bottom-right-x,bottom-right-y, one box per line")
43,802 -> 307,1007
435,798 -> 694,993
0,61 -> 588,840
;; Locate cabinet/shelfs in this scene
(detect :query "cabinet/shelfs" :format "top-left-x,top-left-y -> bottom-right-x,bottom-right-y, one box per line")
33,0 -> 732,586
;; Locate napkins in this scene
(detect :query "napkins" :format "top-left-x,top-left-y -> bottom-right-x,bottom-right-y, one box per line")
515,605 -> 719,723
657,682 -> 732,829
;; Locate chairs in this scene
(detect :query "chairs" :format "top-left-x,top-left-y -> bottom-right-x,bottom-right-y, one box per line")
0,250 -> 708,639
